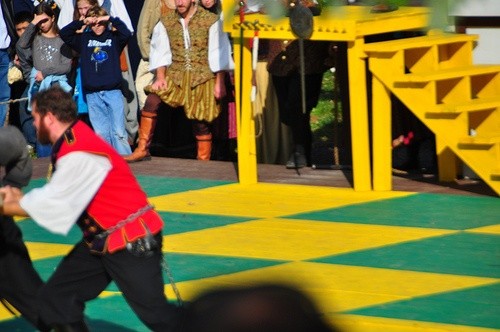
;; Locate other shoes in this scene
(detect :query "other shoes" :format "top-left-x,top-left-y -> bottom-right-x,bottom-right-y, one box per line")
287,152 -> 308,169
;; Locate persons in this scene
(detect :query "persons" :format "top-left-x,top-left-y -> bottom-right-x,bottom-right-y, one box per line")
266,39 -> 333,169
176,281 -> 337,332
0,89 -> 177,332
0,126 -> 46,332
0,0 -> 235,162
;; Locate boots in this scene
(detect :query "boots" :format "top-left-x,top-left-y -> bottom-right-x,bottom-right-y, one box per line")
195,134 -> 212,161
123,111 -> 157,161
